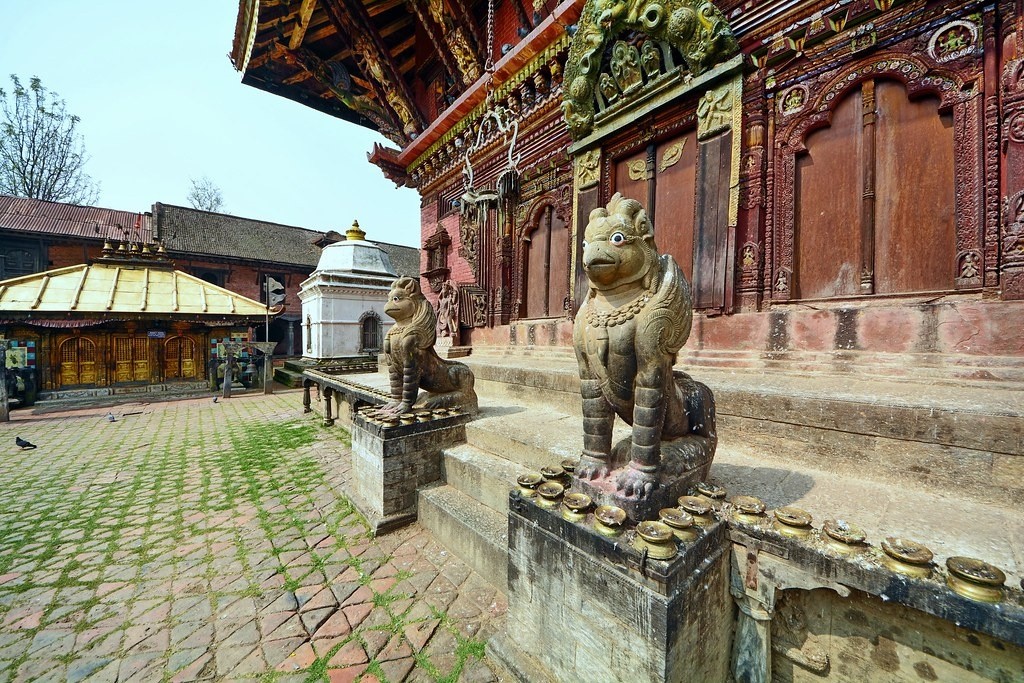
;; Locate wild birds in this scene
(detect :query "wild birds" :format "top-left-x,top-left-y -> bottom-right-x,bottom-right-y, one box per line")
95,224 -> 99,233
16,437 -> 37,449
117,224 -> 122,230
212,395 -> 217,403
122,230 -> 129,239
108,412 -> 115,422
